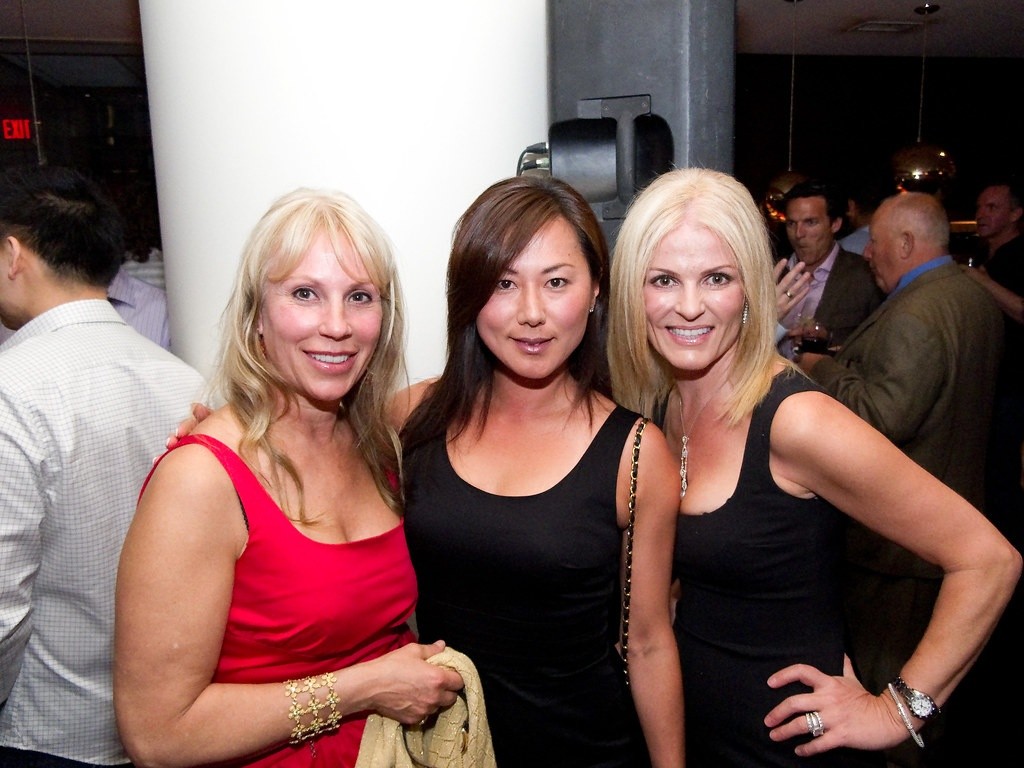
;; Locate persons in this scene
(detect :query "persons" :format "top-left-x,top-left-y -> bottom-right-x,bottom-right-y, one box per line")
607,164 -> 1024,768
111,188 -> 466,767
121,231 -> 165,289
0,259 -> 171,351
768,178 -> 1023,695
159,175 -> 685,768
0,163 -> 210,768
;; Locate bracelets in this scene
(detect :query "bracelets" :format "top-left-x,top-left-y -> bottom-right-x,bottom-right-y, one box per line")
283,671 -> 342,744
887,683 -> 925,747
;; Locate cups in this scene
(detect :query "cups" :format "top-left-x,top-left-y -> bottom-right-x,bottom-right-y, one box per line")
959,256 -> 980,269
802,321 -> 835,355
791,312 -> 814,355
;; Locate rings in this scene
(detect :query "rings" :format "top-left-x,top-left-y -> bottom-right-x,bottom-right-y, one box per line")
784,290 -> 794,299
418,716 -> 429,726
805,710 -> 824,736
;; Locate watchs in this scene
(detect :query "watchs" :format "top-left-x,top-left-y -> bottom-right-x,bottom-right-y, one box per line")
892,675 -> 943,724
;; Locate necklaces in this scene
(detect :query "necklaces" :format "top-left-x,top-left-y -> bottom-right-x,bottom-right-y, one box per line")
678,376 -> 731,502
